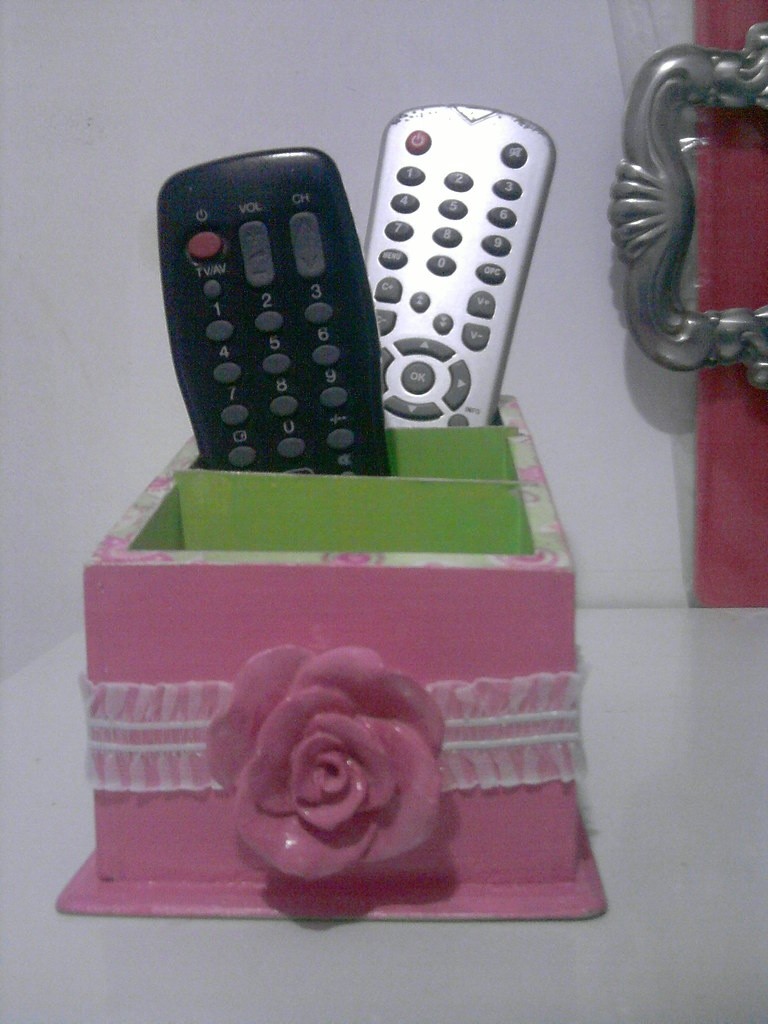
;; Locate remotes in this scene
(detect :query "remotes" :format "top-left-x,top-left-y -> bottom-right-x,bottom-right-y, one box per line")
157,144 -> 391,478
365,105 -> 556,429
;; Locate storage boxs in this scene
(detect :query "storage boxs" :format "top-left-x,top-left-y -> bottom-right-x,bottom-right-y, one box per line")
53,394 -> 608,929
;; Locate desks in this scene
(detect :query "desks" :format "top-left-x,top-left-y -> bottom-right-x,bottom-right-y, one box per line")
0,608 -> 768,1024
